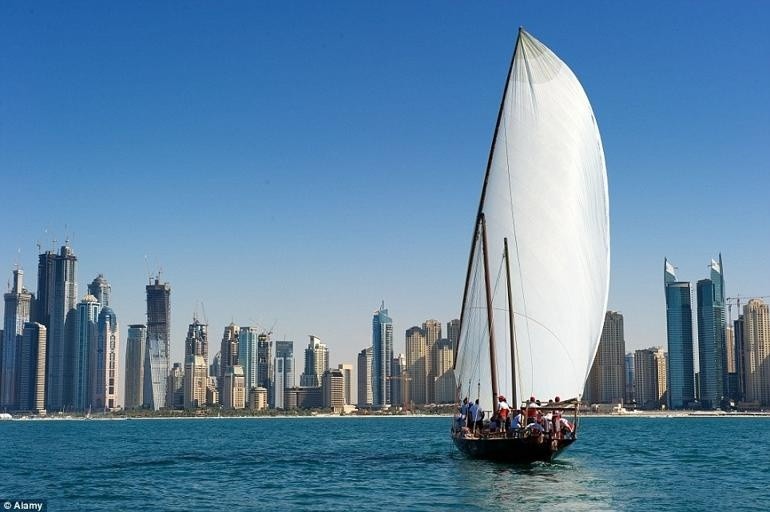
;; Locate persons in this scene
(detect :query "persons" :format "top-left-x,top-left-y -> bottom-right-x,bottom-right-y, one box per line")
452,396 -> 601,444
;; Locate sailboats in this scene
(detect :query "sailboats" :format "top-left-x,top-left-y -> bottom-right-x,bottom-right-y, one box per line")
450,26 -> 611,463
